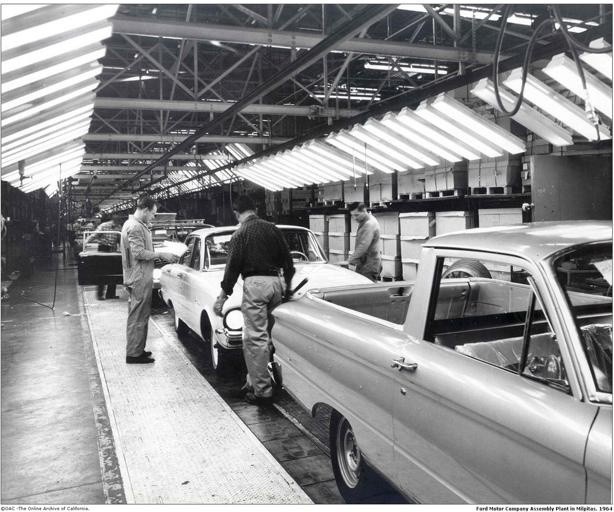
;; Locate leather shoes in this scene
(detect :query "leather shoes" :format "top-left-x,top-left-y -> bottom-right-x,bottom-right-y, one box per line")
126,351 -> 154,363
245,393 -> 273,405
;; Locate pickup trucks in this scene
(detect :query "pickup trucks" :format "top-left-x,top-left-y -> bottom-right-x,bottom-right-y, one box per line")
266,216 -> 612,504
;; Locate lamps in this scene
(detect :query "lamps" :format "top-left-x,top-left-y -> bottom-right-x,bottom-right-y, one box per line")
0,4 -> 613,219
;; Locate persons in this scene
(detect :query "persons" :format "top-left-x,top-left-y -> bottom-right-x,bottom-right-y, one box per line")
212,193 -> 296,405
333,201 -> 383,283
119,197 -> 183,363
80,215 -> 122,300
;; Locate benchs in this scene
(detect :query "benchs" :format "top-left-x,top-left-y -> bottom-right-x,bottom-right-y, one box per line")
454,323 -> 613,394
